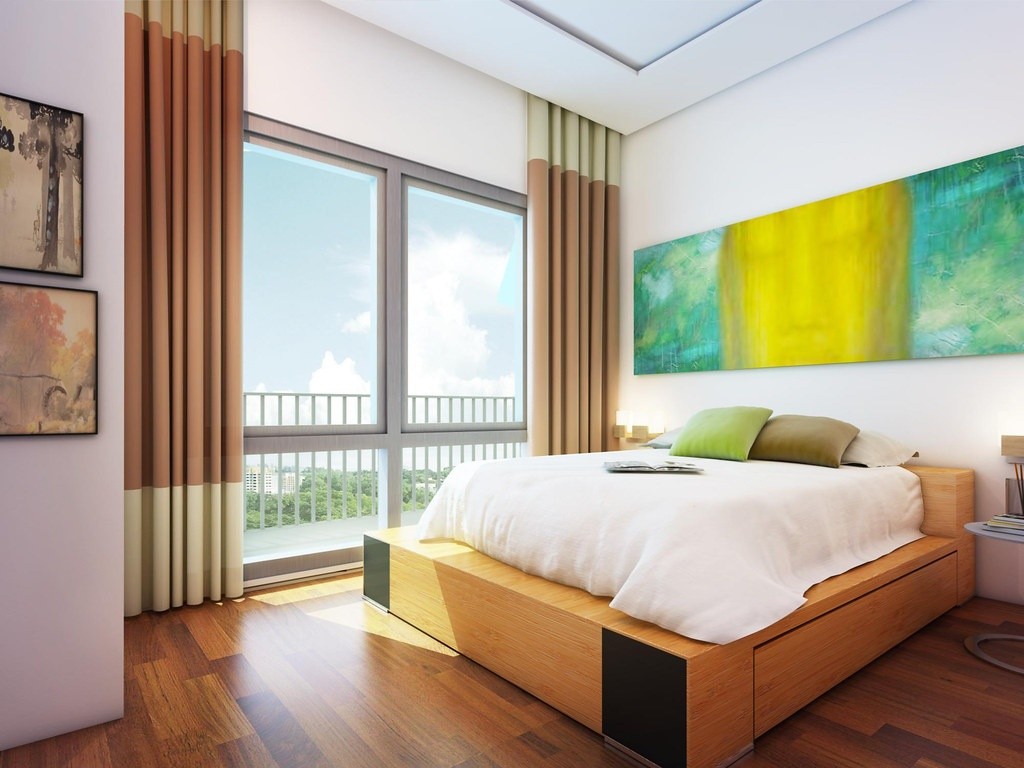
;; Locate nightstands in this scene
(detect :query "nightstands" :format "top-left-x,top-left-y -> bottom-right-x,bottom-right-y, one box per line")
963,520 -> 1024,675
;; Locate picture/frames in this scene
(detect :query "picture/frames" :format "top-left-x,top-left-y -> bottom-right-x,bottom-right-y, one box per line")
0,93 -> 85,278
0,281 -> 100,434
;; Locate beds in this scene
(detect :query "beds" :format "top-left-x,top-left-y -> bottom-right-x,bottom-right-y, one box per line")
363,447 -> 975,768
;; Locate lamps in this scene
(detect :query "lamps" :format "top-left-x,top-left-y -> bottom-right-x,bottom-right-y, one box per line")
995,435 -> 1024,520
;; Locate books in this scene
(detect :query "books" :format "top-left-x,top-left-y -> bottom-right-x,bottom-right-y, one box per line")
604,461 -> 706,472
982,512 -> 1024,538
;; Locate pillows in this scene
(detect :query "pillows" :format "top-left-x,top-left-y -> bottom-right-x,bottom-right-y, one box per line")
749,413 -> 860,468
638,427 -> 685,449
668,406 -> 773,462
840,430 -> 919,468
